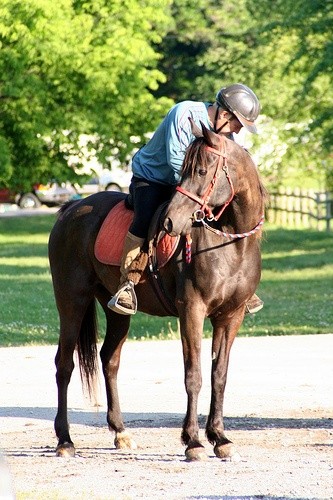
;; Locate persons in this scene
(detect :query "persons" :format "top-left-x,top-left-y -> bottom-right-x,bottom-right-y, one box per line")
110,82 -> 264,312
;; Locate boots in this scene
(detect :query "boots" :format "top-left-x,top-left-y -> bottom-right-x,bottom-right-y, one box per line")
245,295 -> 260,314
117,231 -> 145,309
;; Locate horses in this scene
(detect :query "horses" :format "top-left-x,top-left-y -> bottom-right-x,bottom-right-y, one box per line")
48,117 -> 266,462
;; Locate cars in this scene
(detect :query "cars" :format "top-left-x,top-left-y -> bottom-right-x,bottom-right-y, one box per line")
14,153 -> 131,209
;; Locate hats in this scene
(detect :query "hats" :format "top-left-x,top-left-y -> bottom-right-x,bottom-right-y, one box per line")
215,83 -> 260,134
